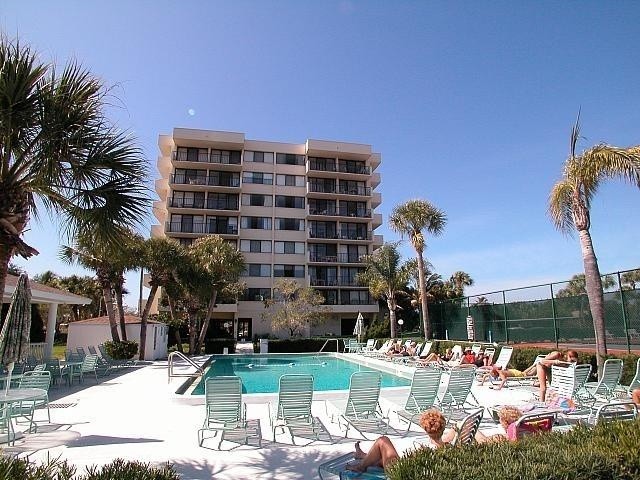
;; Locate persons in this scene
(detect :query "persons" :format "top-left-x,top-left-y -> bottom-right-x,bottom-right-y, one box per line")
489,364 -> 537,391
385,341 -> 417,357
631,388 -> 640,405
454,348 -> 489,368
535,349 -> 578,402
442,403 -> 523,445
418,347 -> 453,363
345,410 -> 457,477
386,340 -> 402,354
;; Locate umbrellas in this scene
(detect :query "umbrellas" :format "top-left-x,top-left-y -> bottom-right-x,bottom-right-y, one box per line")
0,270 -> 34,436
352,313 -> 367,343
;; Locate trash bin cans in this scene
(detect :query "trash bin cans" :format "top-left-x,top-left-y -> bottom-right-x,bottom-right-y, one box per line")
260,339 -> 268,353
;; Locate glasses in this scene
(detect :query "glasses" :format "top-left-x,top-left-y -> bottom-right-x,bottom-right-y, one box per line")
468,350 -> 471,352
479,353 -> 483,355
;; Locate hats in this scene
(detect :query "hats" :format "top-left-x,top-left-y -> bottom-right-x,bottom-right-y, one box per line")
410,342 -> 416,346
464,347 -> 473,353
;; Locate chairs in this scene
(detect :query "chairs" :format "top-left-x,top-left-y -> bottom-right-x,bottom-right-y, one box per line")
573,363 -> 592,408
198,376 -> 249,451
610,359 -> 640,404
594,402 -> 638,425
524,361 -> 576,426
487,403 -> 536,425
268,374 -> 322,447
318,408 -> 485,480
478,410 -> 557,442
392,366 -> 442,439
0,341 -> 140,446
435,363 -> 480,421
343,338 -> 513,386
585,358 -> 623,405
325,371 -> 390,441
